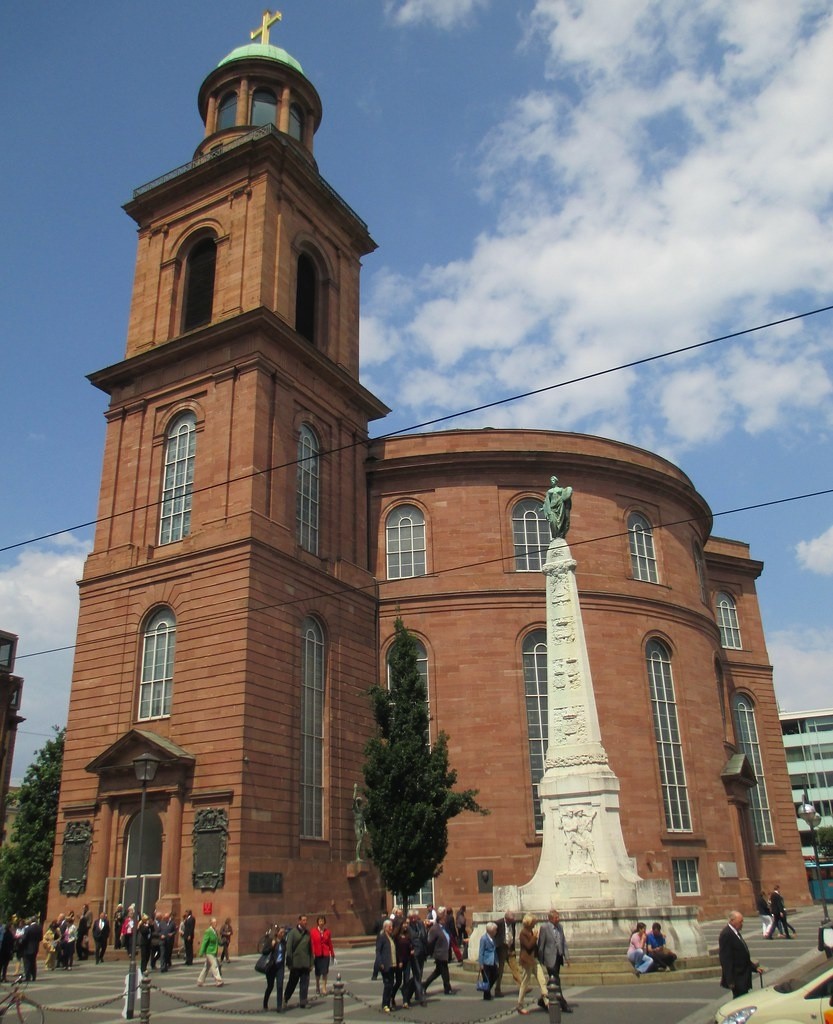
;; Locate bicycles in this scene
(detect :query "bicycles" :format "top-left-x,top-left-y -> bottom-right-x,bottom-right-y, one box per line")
0,972 -> 46,1024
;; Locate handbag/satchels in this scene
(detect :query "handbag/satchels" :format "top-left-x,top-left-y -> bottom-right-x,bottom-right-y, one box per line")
254,948 -> 274,974
219,936 -> 227,946
476,968 -> 490,991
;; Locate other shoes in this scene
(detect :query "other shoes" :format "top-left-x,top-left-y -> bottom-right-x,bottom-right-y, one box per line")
197,982 -> 203,987
316,987 -> 320,994
68,967 -> 71,971
263,1001 -> 268,1009
658,966 -> 670,971
562,1006 -> 573,1013
12,972 -> 19,975
299,1003 -> 312,1008
444,989 -> 454,995
403,1002 -> 408,1008
216,981 -> 223,986
62,966 -> 66,970
277,1008 -> 284,1013
421,1000 -> 427,1006
526,988 -> 532,993
283,998 -> 286,1009
384,1005 -> 391,1013
538,999 -> 545,1007
518,1007 -> 528,1014
495,991 -> 504,997
762,931 -> 796,939
634,969 -> 640,974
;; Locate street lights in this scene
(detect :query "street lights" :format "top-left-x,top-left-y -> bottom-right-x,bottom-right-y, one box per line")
124,749 -> 163,1020
798,777 -> 829,925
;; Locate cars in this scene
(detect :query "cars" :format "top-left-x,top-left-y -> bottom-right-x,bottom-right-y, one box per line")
713,957 -> 833,1024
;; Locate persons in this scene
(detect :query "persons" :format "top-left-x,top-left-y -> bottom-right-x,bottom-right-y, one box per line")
628,923 -> 677,977
261,926 -> 285,1011
479,922 -> 500,1000
219,917 -> 233,963
0,900 -> 195,984
515,912 -> 553,1015
196,917 -> 226,988
493,911 -> 533,998
543,475 -> 566,542
373,902 -> 472,1013
758,885 -> 797,939
282,914 -> 314,1010
718,911 -> 765,999
536,909 -> 573,1013
310,916 -> 334,995
